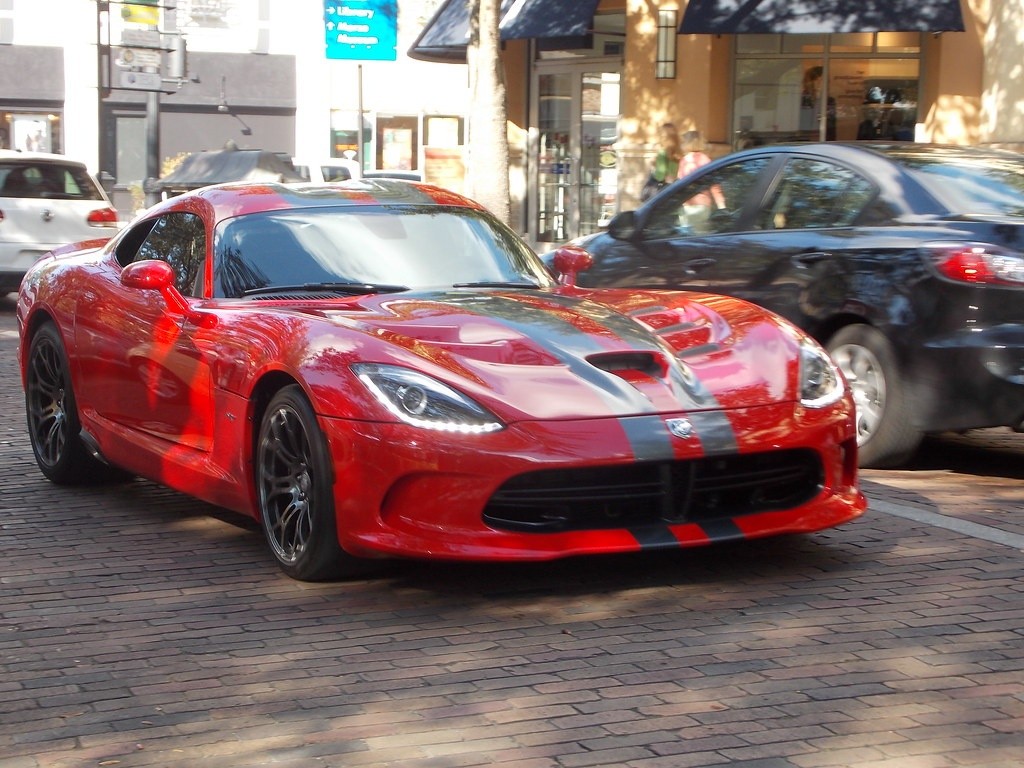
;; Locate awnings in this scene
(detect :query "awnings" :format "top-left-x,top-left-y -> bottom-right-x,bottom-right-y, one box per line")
675,0 -> 963,33
407,0 -> 596,65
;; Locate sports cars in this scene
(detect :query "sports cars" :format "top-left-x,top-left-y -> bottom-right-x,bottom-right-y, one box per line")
16,181 -> 868,585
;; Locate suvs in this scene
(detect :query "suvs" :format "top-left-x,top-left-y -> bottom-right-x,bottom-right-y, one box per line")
0,148 -> 118,300
291,157 -> 362,184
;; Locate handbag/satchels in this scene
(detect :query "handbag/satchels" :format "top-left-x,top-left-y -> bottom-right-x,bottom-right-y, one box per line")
640,178 -> 670,202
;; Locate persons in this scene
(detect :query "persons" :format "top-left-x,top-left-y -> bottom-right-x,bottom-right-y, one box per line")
648,123 -> 679,185
856,108 -> 882,140
677,132 -> 726,232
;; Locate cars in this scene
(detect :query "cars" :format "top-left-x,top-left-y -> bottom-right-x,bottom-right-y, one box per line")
536,139 -> 1024,468
